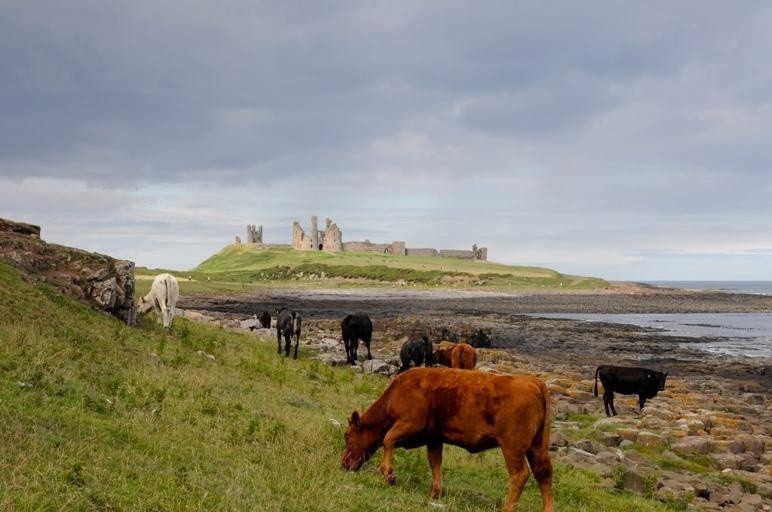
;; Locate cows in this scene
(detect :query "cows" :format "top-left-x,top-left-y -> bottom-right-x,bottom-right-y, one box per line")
136,271 -> 181,330
432,342 -> 479,368
255,310 -> 272,329
593,364 -> 669,417
398,333 -> 430,370
339,313 -> 374,364
273,306 -> 302,360
338,365 -> 556,512
